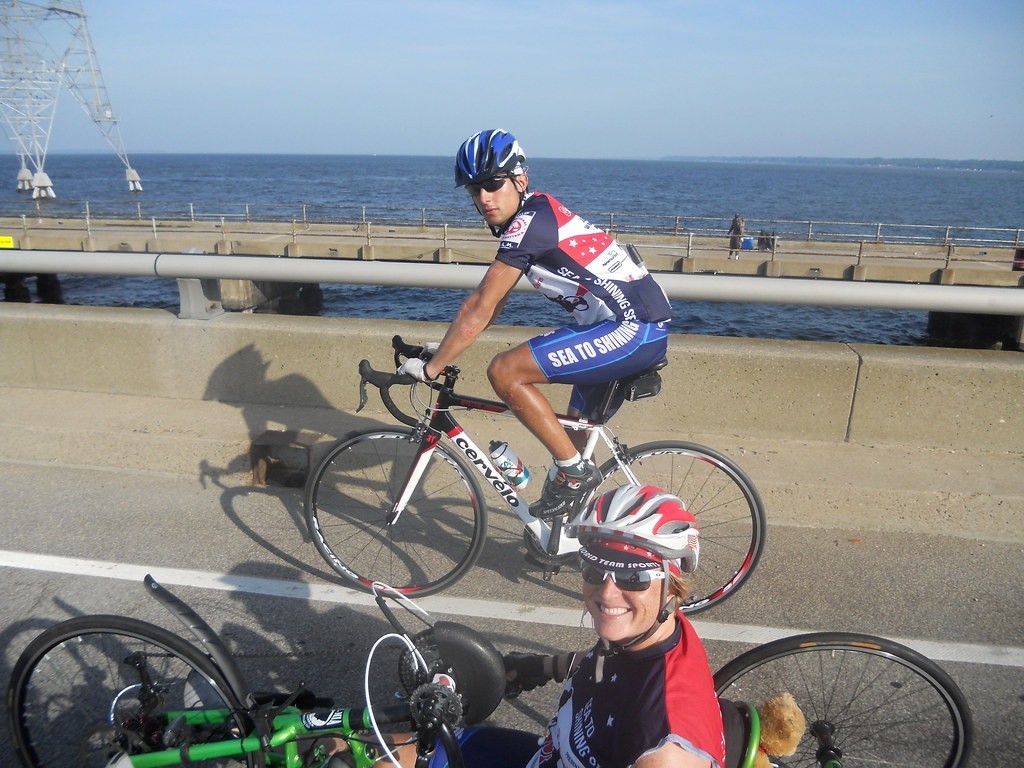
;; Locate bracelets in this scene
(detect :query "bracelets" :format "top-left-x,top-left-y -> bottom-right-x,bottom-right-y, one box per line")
423,362 -> 440,381
566,651 -> 574,680
552,654 -> 564,684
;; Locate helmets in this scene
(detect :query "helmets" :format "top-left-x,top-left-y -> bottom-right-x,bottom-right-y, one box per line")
454,129 -> 526,187
565,484 -> 701,574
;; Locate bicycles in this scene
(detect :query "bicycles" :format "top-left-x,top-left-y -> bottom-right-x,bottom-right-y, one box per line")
303,335 -> 767,616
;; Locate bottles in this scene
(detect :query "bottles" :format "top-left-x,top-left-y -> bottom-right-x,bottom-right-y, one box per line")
489,440 -> 531,491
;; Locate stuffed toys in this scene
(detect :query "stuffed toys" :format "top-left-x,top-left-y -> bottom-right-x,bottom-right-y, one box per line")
751,691 -> 807,768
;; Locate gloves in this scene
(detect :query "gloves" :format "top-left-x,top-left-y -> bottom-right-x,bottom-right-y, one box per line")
398,342 -> 441,384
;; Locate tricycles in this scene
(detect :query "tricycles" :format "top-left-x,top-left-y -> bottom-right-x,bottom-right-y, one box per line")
7,570 -> 970,768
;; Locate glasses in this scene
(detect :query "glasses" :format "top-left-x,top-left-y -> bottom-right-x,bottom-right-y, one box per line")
578,558 -> 667,591
465,174 -> 517,196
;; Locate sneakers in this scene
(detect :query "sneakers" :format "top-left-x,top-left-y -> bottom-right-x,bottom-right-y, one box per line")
529,458 -> 604,519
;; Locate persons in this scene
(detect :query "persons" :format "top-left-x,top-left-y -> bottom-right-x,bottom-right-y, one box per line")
307,483 -> 726,768
397,126 -> 673,519
727,212 -> 745,260
